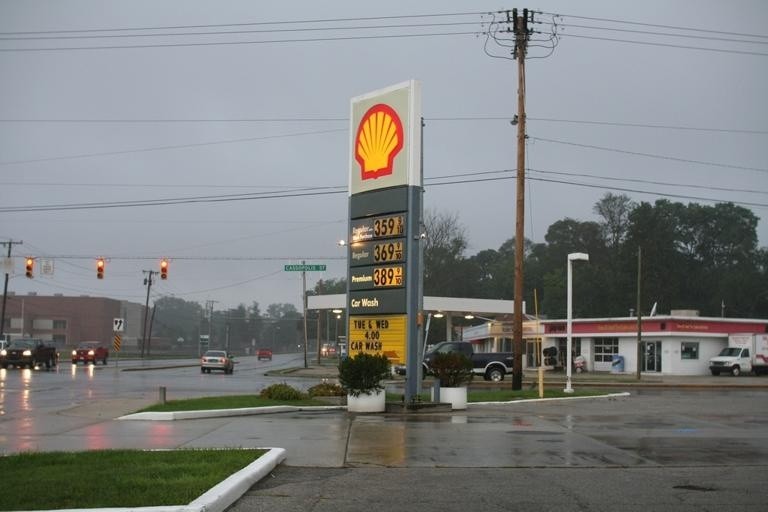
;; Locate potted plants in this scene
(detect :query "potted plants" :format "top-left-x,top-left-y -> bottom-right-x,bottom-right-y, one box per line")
422,350 -> 471,412
337,353 -> 390,416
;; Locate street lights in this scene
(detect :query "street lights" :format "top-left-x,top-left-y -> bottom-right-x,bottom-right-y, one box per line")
338,240 -> 348,247
563,251 -> 590,395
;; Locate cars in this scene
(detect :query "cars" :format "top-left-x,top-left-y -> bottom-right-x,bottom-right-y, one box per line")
256,349 -> 272,360
320,343 -> 339,358
199,349 -> 235,375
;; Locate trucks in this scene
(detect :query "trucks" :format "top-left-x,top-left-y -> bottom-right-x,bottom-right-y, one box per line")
707,332 -> 767,376
0,333 -> 31,350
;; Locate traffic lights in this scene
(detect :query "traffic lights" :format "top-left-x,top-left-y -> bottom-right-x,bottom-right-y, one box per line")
160,261 -> 167,280
96,260 -> 104,279
24,258 -> 34,279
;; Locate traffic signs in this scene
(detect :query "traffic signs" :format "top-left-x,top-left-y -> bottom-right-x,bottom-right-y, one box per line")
284,265 -> 328,271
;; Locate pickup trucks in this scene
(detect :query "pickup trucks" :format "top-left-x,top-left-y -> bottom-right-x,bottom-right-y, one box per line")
0,338 -> 56,369
394,341 -> 515,385
72,341 -> 109,366
